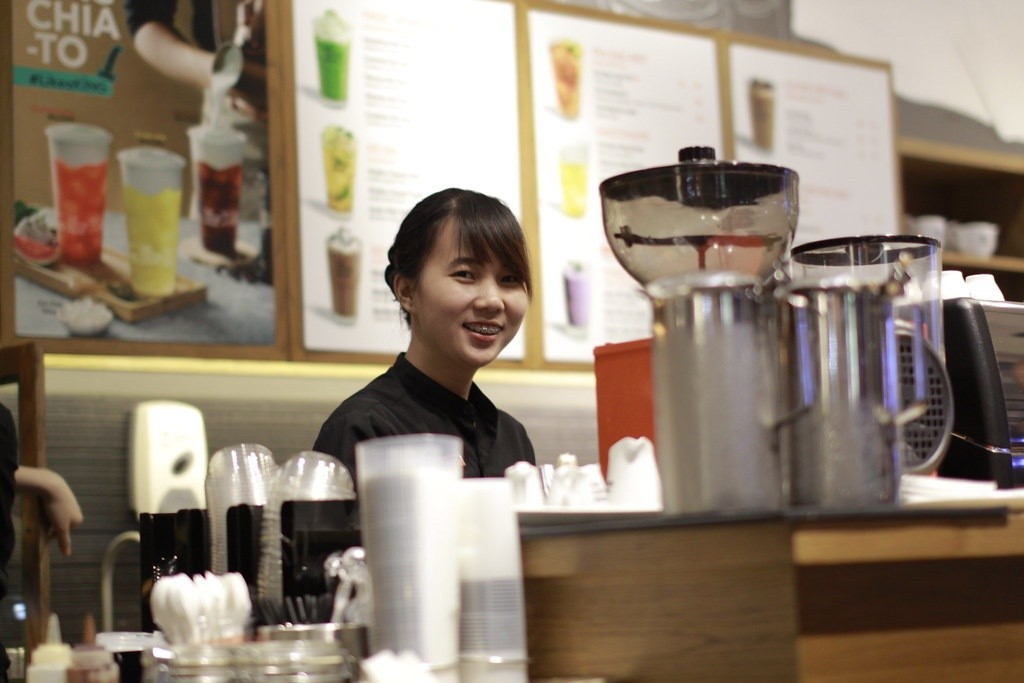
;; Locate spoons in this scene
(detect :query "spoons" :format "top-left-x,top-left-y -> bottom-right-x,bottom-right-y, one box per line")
151,573 -> 250,648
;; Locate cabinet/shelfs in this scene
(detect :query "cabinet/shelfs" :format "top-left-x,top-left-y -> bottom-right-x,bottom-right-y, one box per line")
899,137 -> 1024,284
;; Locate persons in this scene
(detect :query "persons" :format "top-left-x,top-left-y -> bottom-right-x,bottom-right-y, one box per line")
123,0 -> 217,92
312,188 -> 538,493
0,406 -> 82,567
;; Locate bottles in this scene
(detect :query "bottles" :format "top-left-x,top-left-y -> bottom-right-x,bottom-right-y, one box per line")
68,617 -> 119,683
26,614 -> 68,683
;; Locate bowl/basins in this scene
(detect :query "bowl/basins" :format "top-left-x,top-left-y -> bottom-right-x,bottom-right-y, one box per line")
599,146 -> 798,283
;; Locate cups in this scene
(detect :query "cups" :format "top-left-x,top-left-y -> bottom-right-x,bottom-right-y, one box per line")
207,443 -> 354,606
645,274 -> 811,513
327,236 -> 362,318
46,123 -> 114,264
356,434 -> 528,683
565,271 -> 591,325
790,286 -> 953,512
561,158 -> 588,215
324,126 -> 358,214
791,235 -> 945,478
317,38 -> 350,101
552,45 -> 581,119
187,126 -> 246,253
752,82 -> 773,147
118,147 -> 186,298
